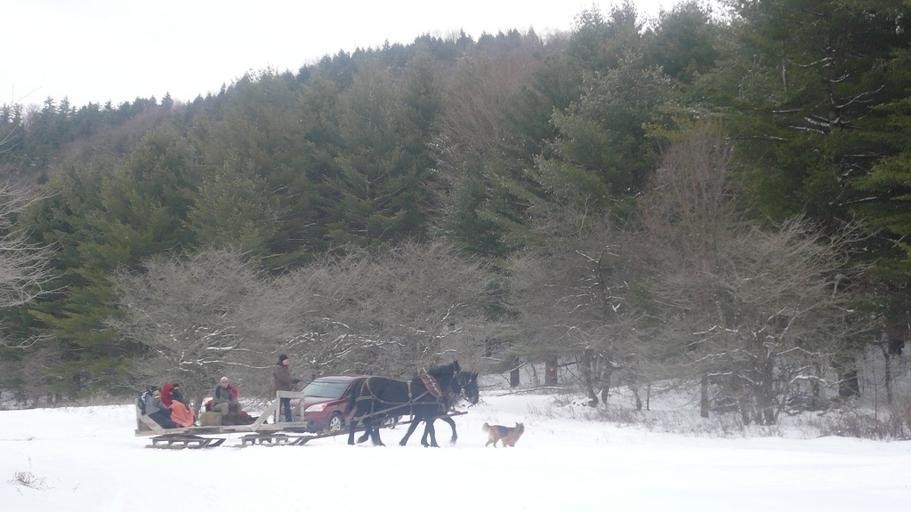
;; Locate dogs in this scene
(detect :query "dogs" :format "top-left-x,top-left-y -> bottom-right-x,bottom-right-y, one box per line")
483,422 -> 524,448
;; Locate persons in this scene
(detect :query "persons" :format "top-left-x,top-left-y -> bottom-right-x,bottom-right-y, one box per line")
210,375 -> 240,425
138,383 -> 196,428
272,355 -> 301,422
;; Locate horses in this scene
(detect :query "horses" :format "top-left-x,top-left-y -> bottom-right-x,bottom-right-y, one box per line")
341,359 -> 479,447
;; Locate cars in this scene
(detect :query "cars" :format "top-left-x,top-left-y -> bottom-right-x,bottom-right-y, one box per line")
273,375 -> 386,436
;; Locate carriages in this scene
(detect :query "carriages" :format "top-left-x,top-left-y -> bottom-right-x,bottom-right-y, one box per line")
134,352 -> 479,451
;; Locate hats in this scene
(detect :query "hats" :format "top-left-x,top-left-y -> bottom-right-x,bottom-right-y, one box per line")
279,354 -> 288,361
